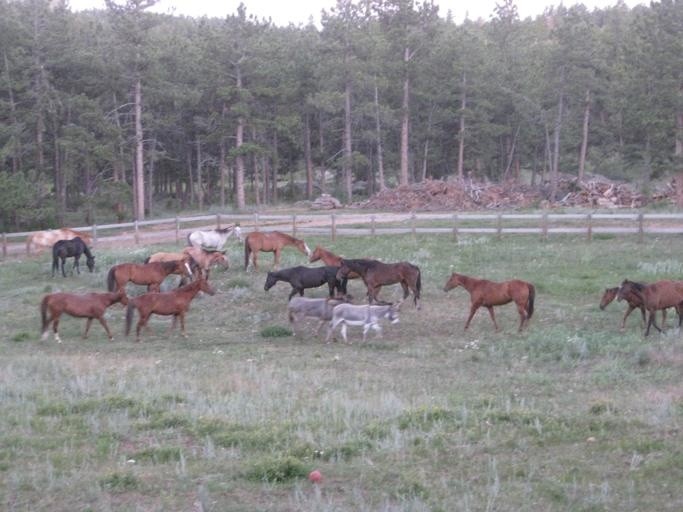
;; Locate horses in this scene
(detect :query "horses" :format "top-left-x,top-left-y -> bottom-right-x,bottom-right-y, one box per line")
617,279 -> 683,336
444,272 -> 535,333
27,222 -> 245,344
243,231 -> 311,271
264,245 -> 425,344
600,287 -> 667,333
